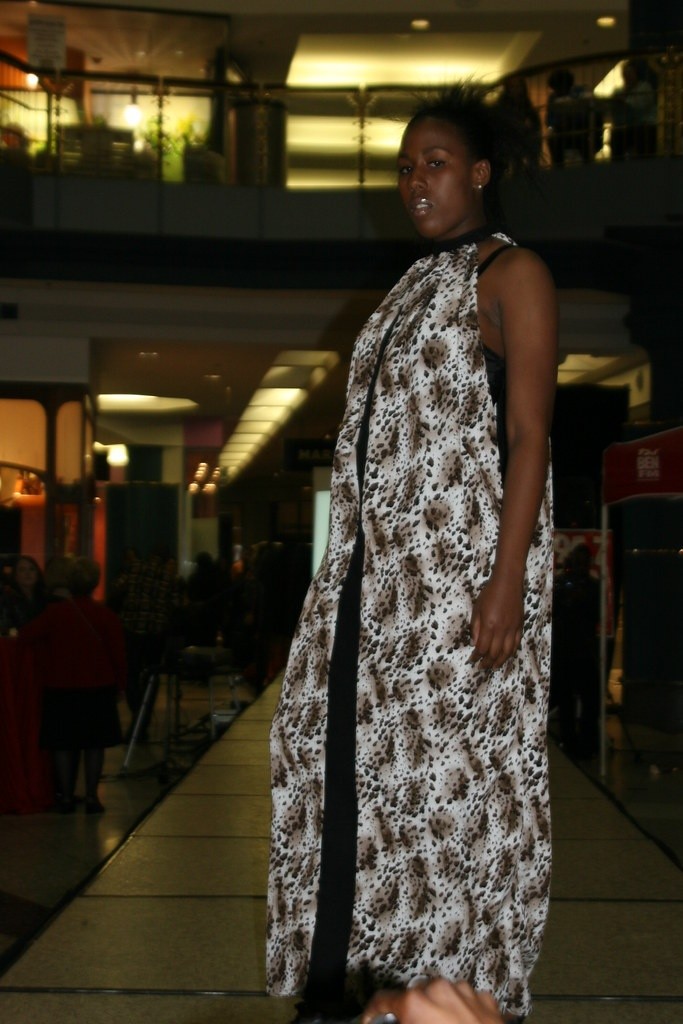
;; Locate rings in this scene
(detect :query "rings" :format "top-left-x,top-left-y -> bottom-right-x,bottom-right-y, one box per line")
406,975 -> 426,988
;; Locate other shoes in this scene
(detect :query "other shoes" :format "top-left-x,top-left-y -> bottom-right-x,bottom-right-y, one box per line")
62,801 -> 76,814
85,802 -> 105,814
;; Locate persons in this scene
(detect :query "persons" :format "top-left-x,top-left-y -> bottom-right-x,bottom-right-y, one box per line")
348,974 -> 513,1024
264,87 -> 559,1024
17,555 -> 129,815
0,538 -> 280,746
542,542 -> 602,753
